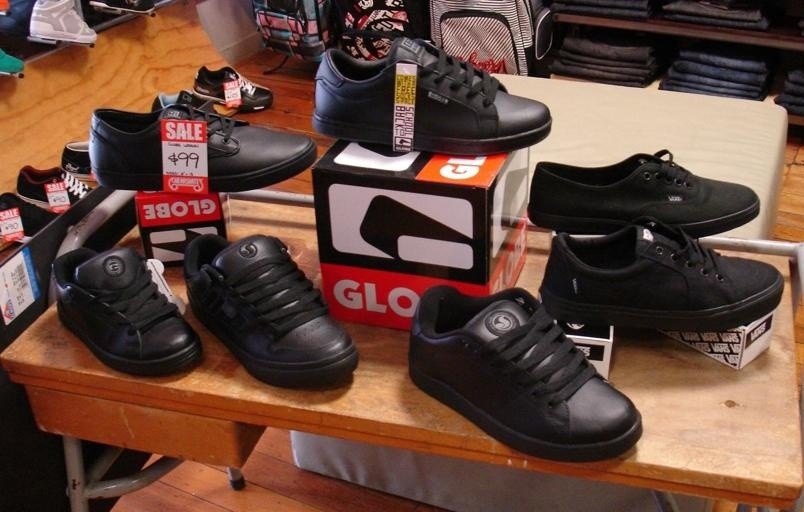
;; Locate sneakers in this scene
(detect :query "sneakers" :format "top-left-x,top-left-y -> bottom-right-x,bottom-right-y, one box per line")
528,149 -> 760,236
51,246 -> 202,377
0,192 -> 56,244
29,0 -> 97,45
63,143 -> 98,181
543,217 -> 784,333
192,65 -> 273,110
89,0 -> 156,16
185,233 -> 359,388
0,47 -> 24,75
312,37 -> 551,155
409,284 -> 642,463
89,104 -> 317,191
16,165 -> 91,209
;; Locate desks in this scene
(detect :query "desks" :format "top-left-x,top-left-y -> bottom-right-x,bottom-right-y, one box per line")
0,189 -> 803,512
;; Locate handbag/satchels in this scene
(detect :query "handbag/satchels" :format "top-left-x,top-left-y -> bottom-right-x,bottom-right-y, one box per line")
430,0 -> 554,75
250,1 -> 330,65
324,0 -> 430,61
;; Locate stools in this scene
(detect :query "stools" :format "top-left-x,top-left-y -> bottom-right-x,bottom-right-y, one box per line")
487,72 -> 790,243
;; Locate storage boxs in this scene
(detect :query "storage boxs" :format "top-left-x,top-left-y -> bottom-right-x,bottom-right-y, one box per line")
310,141 -> 527,333
